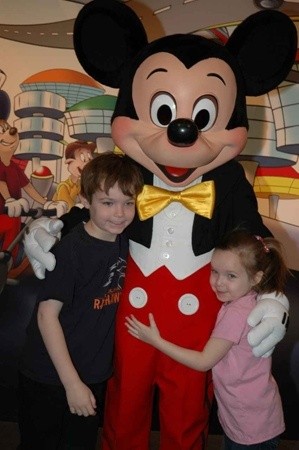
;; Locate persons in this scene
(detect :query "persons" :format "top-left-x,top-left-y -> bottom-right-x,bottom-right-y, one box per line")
19,150 -> 144,450
125,222 -> 286,450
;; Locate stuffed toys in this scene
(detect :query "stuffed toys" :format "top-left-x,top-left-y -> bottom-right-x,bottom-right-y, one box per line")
22,0 -> 298,450
